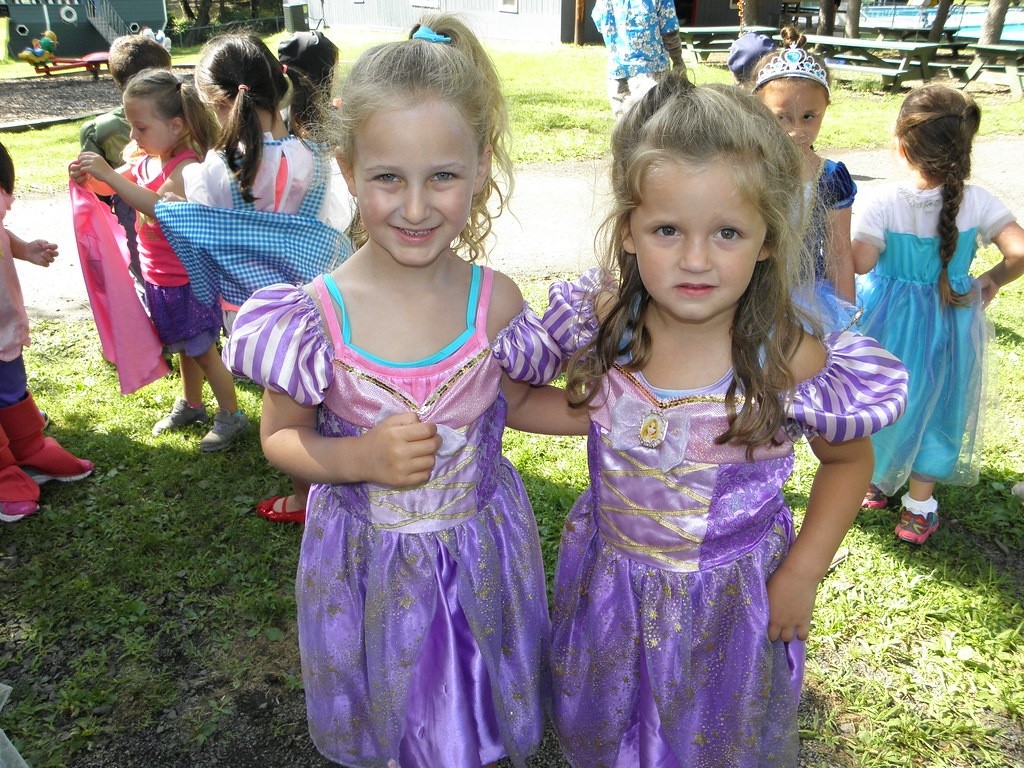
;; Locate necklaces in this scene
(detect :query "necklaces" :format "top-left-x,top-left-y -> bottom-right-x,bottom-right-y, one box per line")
613,363 -> 747,447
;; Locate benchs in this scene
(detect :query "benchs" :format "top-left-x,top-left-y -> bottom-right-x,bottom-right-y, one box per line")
780,11 -> 815,28
682,42 -> 1024,93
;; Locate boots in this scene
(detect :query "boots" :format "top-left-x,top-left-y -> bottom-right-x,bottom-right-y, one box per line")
0,390 -> 95,485
0,424 -> 40,522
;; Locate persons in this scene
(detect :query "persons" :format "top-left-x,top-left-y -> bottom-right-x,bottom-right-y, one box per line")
590,0 -> 688,124
538,67 -> 910,768
221,14 -> 591,767
67,31 -> 356,522
0,141 -> 95,525
729,25 -> 1024,544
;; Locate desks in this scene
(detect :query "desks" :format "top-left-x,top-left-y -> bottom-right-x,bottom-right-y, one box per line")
677,26 -> 782,64
956,44 -> 1024,94
873,27 -> 962,58
782,6 -> 847,28
772,32 -> 941,93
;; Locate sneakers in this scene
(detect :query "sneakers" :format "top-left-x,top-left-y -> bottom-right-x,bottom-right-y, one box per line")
861,483 -> 887,509
895,506 -> 938,543
200,407 -> 251,451
151,398 -> 207,435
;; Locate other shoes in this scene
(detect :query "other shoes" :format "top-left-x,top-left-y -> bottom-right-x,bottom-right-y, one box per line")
38,409 -> 51,429
256,496 -> 306,524
